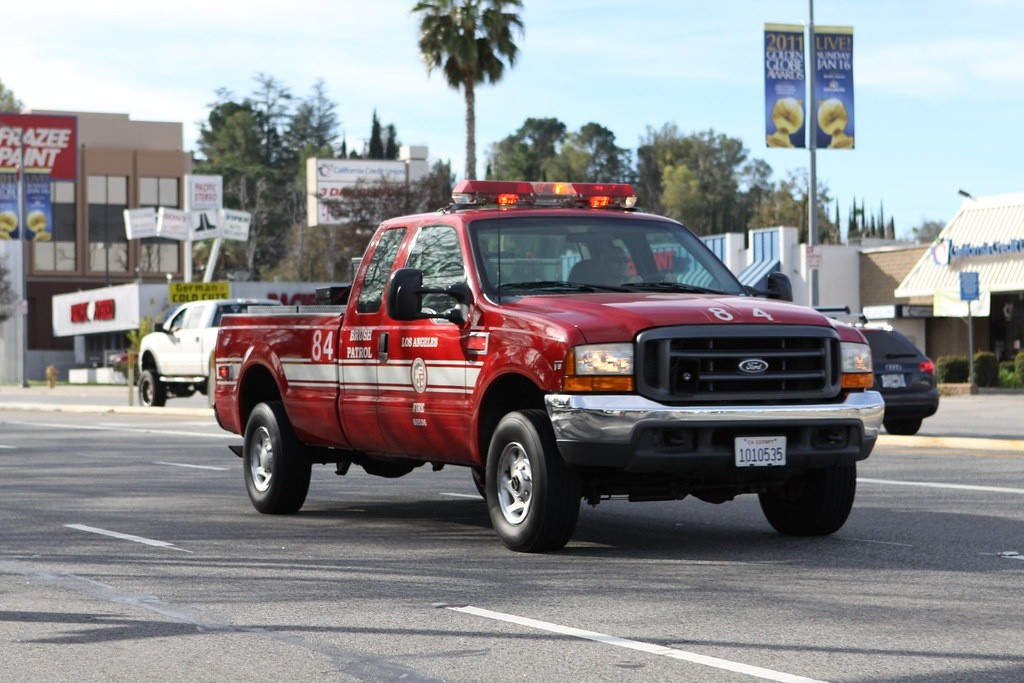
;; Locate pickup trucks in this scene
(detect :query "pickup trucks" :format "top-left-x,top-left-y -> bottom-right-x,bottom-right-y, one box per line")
211,180 -> 887,552
137,299 -> 285,407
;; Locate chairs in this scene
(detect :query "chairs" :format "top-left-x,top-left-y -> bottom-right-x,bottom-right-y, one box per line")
568,258 -> 600,286
449,240 -> 510,289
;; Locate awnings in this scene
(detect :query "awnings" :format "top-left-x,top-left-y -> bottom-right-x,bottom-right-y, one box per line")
894,203 -> 1024,299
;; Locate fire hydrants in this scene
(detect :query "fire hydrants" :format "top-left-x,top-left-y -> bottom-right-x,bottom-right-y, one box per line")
45,365 -> 59,389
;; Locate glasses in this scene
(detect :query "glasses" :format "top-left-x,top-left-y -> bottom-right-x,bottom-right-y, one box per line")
607,257 -> 631,264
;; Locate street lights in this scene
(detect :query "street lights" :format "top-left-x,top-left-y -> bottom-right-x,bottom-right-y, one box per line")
958,189 -> 1023,245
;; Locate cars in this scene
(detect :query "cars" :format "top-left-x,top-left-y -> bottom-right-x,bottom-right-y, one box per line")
853,328 -> 939,435
107,353 -> 138,367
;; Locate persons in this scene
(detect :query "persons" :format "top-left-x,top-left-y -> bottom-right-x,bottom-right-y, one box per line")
422,240 -> 510,310
601,247 -> 643,285
